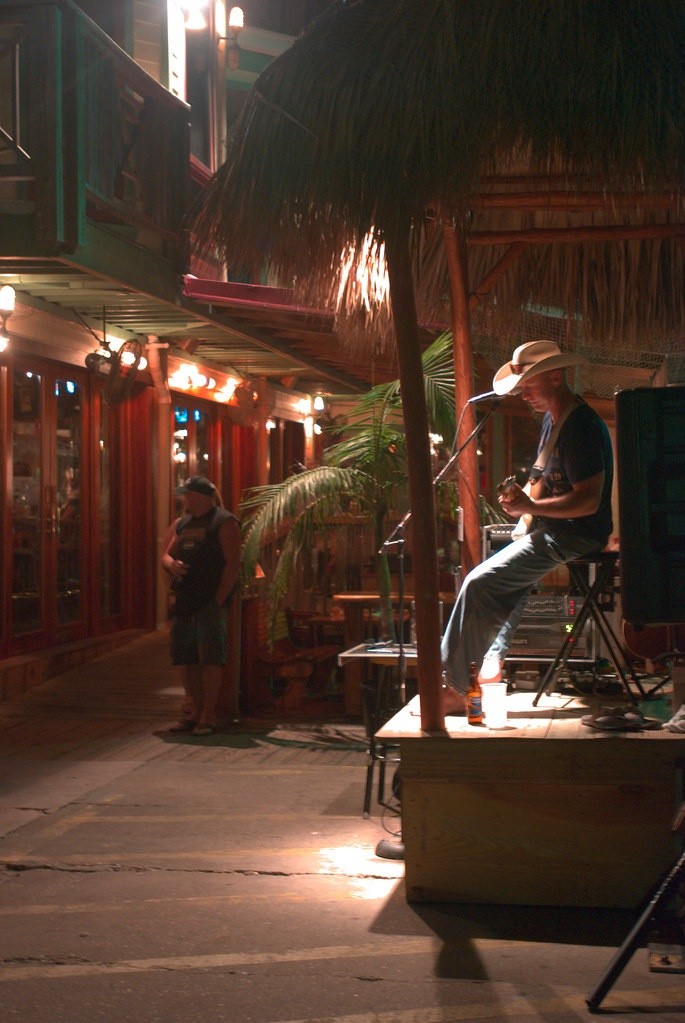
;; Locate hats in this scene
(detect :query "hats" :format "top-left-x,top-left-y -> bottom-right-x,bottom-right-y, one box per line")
174,476 -> 217,498
493,339 -> 583,395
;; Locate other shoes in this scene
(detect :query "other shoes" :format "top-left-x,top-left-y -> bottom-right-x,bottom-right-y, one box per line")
441,674 -> 470,717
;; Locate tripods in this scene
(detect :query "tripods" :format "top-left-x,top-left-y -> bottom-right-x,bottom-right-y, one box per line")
362,398 -> 504,818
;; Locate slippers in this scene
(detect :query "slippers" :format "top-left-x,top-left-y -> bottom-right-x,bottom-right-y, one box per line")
191,722 -> 217,737
169,719 -> 199,732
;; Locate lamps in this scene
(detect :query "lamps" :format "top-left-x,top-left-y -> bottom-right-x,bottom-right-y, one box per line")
0,285 -> 16,352
216,6 -> 244,70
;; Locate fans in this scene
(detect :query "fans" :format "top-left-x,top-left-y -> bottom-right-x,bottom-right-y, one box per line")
84,337 -> 143,409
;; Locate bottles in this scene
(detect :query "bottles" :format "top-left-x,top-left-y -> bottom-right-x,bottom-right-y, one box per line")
465,661 -> 483,725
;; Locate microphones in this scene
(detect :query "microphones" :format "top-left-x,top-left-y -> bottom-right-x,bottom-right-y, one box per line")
468,386 -> 522,404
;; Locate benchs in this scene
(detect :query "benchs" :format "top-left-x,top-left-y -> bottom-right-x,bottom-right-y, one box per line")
244,599 -> 344,699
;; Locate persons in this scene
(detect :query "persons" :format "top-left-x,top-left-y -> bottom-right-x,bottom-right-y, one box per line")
161,475 -> 242,736
421,339 -> 613,715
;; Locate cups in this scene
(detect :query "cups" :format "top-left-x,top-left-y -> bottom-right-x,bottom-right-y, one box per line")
478,683 -> 509,729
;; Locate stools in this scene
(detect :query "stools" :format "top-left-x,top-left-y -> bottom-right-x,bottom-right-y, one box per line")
534,551 -> 650,708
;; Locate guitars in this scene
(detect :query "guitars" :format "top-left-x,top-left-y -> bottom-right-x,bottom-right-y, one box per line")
495,474 -> 536,535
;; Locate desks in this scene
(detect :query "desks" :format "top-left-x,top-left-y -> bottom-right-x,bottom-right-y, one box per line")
333,592 -> 459,716
308,614 -> 411,646
